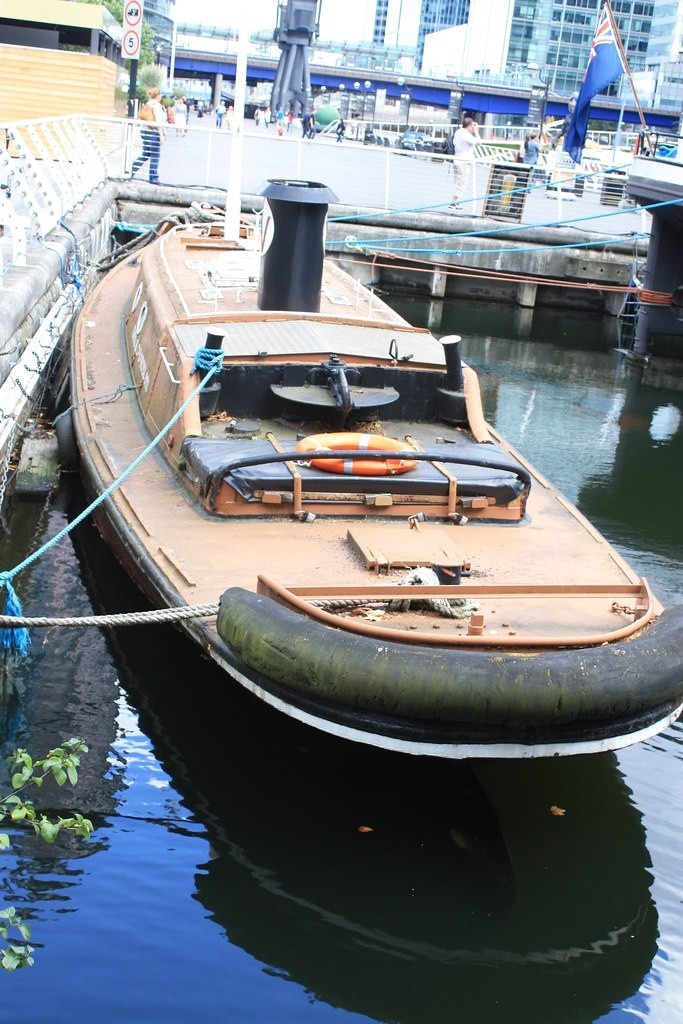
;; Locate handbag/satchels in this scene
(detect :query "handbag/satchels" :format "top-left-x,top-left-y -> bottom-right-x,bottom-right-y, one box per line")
515,154 -> 520,164
140,101 -> 158,130
337,127 -> 342,135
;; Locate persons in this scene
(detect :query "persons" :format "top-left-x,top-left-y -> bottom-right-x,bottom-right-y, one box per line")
442,115 -> 482,209
131,87 -> 165,183
254,105 -> 318,140
162,94 -> 234,137
335,118 -> 346,142
518,133 -> 542,164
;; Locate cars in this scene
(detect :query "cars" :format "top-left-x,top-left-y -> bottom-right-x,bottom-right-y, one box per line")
397,130 -> 438,159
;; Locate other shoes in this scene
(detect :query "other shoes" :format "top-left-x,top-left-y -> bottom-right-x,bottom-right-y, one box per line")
448,203 -> 461,210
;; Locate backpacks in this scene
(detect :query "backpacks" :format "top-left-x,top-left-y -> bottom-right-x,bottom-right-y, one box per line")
442,134 -> 454,163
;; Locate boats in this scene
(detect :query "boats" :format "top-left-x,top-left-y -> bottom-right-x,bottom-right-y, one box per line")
53,178 -> 683,762
59,479 -> 664,1024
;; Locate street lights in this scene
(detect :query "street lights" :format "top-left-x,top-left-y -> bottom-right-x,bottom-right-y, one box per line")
364,80 -> 379,132
338,83 -> 349,122
352,81 -> 364,124
526,61 -> 553,165
396,76 -> 413,130
446,71 -> 465,128
319,85 -> 327,107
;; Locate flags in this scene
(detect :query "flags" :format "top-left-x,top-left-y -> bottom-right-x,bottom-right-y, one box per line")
566,2 -> 629,165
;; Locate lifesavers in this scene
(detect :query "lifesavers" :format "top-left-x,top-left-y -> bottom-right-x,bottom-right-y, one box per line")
292,431 -> 418,476
